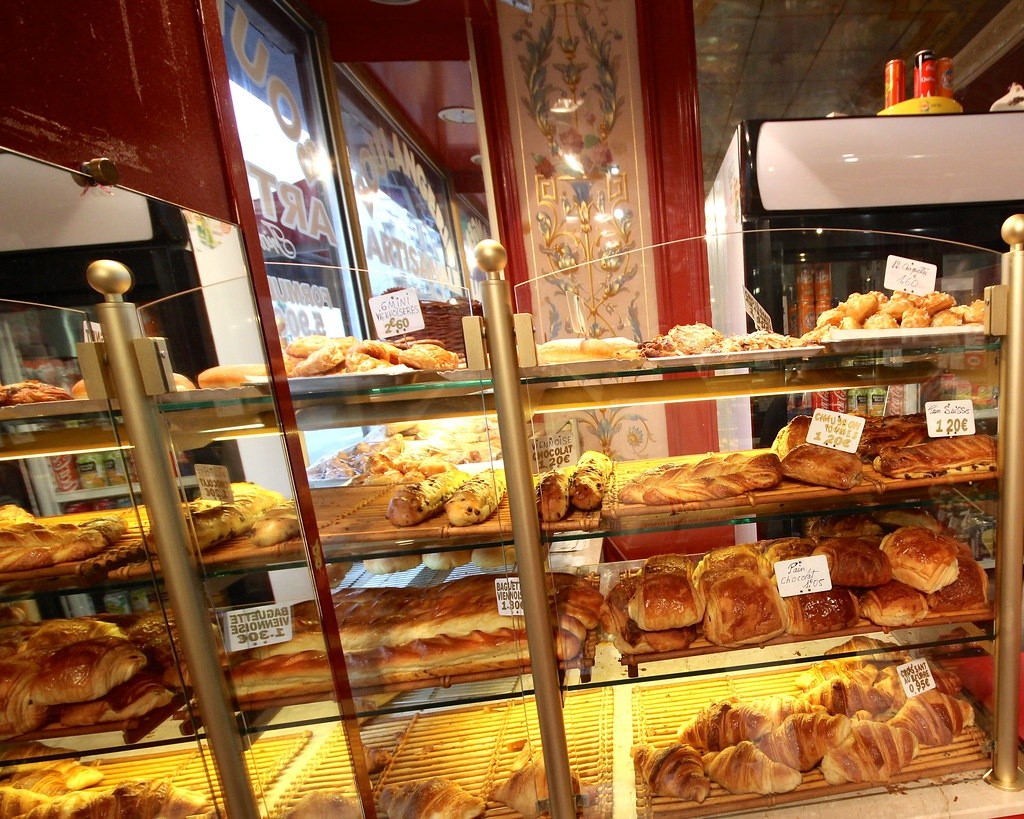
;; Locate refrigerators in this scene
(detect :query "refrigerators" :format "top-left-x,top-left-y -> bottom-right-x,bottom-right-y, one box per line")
699,109 -> 1024,544
0,147 -> 316,618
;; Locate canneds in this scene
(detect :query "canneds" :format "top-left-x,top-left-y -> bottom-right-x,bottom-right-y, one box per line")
884,49 -> 953,108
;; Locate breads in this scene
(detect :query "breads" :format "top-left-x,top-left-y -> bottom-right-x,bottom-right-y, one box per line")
0,289 -> 996,819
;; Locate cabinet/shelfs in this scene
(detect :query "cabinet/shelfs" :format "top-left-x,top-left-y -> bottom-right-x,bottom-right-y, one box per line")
0,214 -> 1024,819
